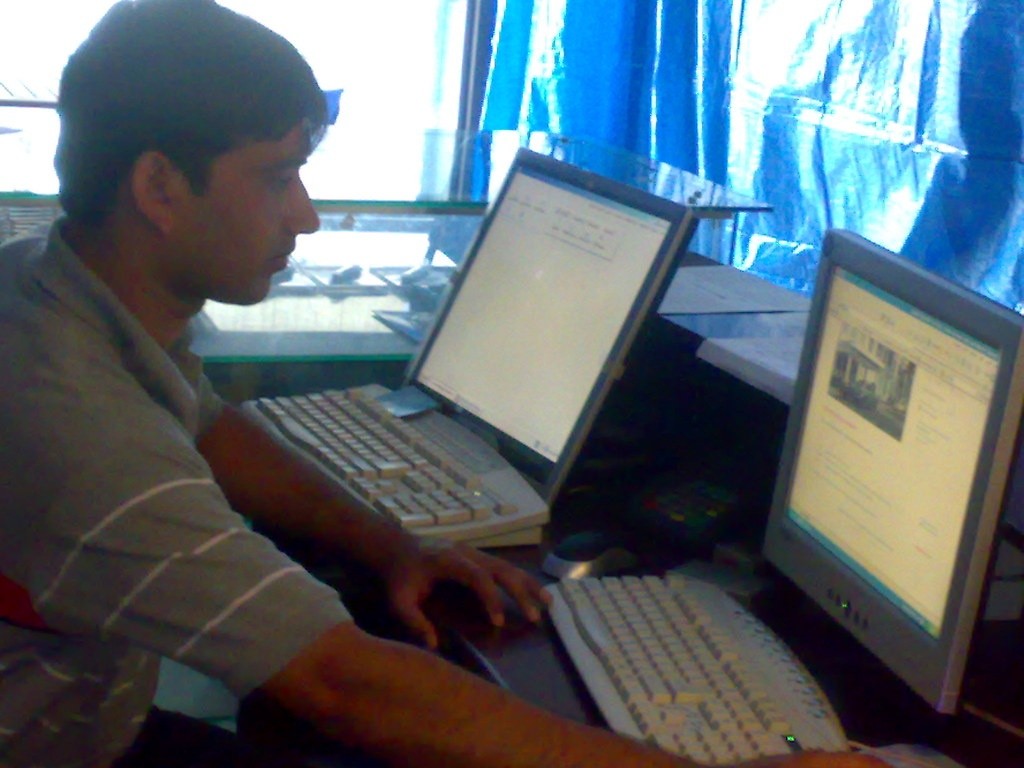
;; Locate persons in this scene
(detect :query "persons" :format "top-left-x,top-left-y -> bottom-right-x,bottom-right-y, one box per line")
0,0 -> 889,768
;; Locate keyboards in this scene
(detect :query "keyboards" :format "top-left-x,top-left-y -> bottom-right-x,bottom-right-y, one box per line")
542,575 -> 851,764
232,382 -> 550,548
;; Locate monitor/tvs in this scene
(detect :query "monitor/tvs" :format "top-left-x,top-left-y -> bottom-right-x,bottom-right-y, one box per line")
398,148 -> 699,510
765,230 -> 1024,712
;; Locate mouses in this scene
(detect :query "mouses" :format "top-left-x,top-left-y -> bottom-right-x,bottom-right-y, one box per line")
541,530 -> 641,580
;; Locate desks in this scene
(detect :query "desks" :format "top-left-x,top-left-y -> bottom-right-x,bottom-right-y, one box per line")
334,528 -> 1024,768
